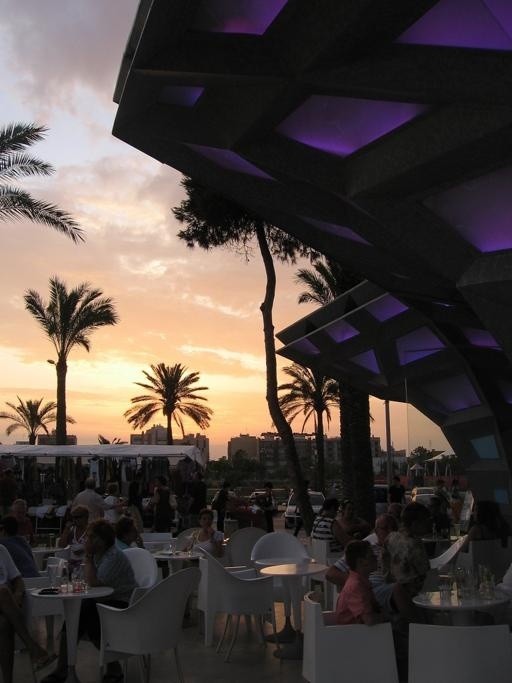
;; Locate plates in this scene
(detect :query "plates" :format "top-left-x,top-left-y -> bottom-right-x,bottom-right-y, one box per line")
158,550 -> 181,554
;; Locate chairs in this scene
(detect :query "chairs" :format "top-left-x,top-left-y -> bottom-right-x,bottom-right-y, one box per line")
297,589 -> 401,681
206,545 -> 285,663
92,581 -> 188,680
57,522 -> 509,680
407,620 -> 509,681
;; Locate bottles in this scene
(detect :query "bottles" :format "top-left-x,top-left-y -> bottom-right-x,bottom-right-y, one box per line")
480,563 -> 496,599
60,560 -> 74,584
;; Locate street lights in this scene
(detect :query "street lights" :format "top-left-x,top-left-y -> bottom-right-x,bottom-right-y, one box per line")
260,446 -> 266,471
313,446 -> 319,491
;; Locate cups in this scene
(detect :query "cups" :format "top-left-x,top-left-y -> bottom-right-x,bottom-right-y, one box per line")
169,537 -> 177,556
80,574 -> 89,592
186,543 -> 193,555
60,584 -> 68,592
453,556 -> 478,596
435,562 -> 456,592
71,573 -> 82,592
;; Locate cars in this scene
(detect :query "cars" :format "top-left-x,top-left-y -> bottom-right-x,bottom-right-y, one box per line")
404,486 -> 448,515
283,489 -> 327,529
376,483 -> 404,515
249,488 -> 278,513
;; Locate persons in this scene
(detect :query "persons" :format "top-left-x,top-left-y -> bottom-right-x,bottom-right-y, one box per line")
427,479 -> 463,538
262,481 -> 274,533
293,480 -> 311,536
473,562 -> 512,633
459,500 -> 512,553
387,477 -> 407,507
211,481 -> 232,533
43,478 -> 80,517
2,499 -> 49,681
59,479 -> 146,580
231,488 -> 241,500
50,520 -> 140,681
127,460 -> 208,532
2,468 -> 19,511
311,498 -> 430,682
177,508 -> 225,618
